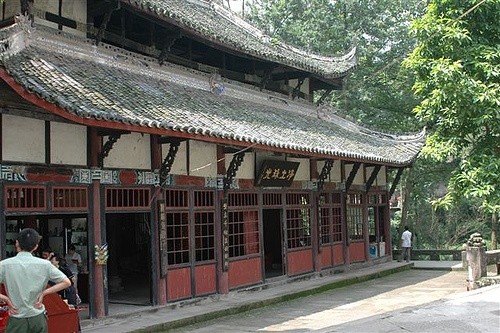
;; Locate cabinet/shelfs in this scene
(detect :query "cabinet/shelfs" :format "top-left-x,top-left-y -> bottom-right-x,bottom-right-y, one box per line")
5,218 -> 89,304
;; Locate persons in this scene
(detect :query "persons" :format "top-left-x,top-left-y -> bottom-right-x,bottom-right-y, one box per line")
0,228 -> 71,333
33,245 -> 74,300
64,245 -> 82,305
396,226 -> 412,263
